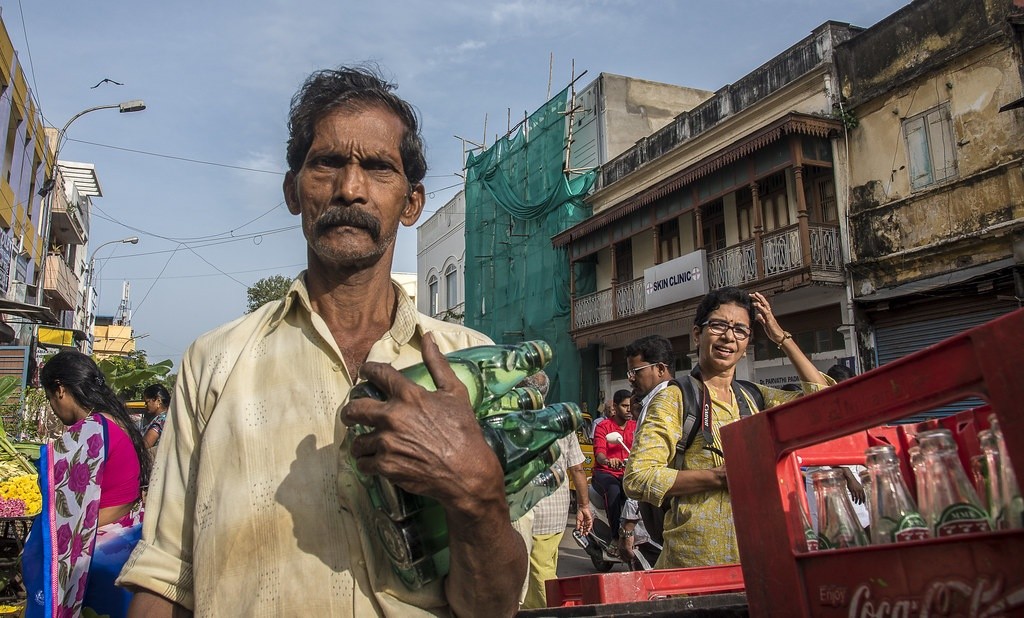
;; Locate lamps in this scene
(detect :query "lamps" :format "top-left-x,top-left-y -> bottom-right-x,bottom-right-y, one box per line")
38,179 -> 56,197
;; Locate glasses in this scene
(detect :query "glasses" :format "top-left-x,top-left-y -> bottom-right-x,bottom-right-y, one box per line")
625,362 -> 669,378
699,319 -> 752,340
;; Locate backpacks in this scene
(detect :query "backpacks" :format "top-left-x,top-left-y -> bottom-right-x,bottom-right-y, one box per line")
635,374 -> 765,548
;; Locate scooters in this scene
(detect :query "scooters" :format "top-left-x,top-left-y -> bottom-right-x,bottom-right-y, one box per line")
572,430 -> 668,572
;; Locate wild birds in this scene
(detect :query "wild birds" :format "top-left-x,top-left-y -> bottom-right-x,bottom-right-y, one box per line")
90,79 -> 124,88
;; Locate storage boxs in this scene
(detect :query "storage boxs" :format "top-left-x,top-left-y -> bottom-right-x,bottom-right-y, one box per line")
544,563 -> 747,608
719,307 -> 1024,618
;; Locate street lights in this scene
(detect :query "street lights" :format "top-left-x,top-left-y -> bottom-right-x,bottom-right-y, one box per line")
82,237 -> 139,354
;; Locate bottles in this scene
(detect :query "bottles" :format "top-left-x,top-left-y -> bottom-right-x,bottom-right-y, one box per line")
799,412 -> 1024,551
343,338 -> 584,592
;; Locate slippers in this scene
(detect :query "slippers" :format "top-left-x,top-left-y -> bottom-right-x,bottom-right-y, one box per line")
607,542 -> 620,558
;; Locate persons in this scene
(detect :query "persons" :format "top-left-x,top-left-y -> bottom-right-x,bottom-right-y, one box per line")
580,333 -> 675,569
15,349 -> 172,618
113,64 -> 538,618
621,286 -> 841,570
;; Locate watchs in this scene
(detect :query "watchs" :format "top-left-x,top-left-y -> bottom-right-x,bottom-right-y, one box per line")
777,331 -> 792,350
617,525 -> 635,539
